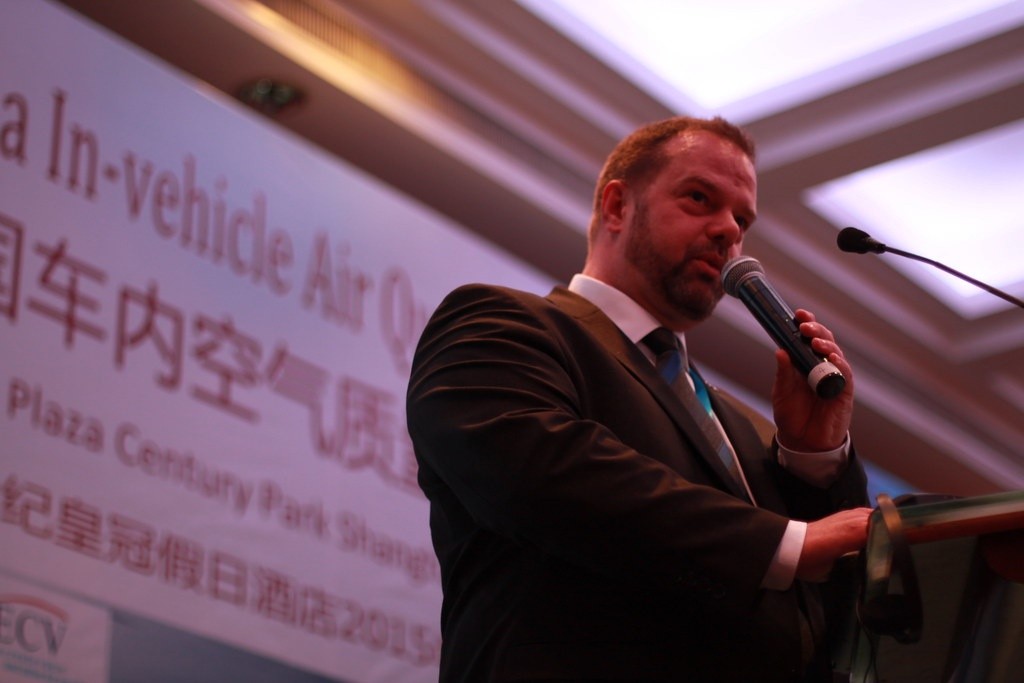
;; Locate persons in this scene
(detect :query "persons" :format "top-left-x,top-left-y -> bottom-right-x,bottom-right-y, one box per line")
406,115 -> 875,683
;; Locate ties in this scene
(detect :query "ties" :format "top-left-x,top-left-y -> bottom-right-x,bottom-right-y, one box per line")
643,326 -> 688,403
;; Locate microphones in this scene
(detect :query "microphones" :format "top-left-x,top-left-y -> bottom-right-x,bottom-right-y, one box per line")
836,226 -> 1024,309
721,256 -> 846,398
